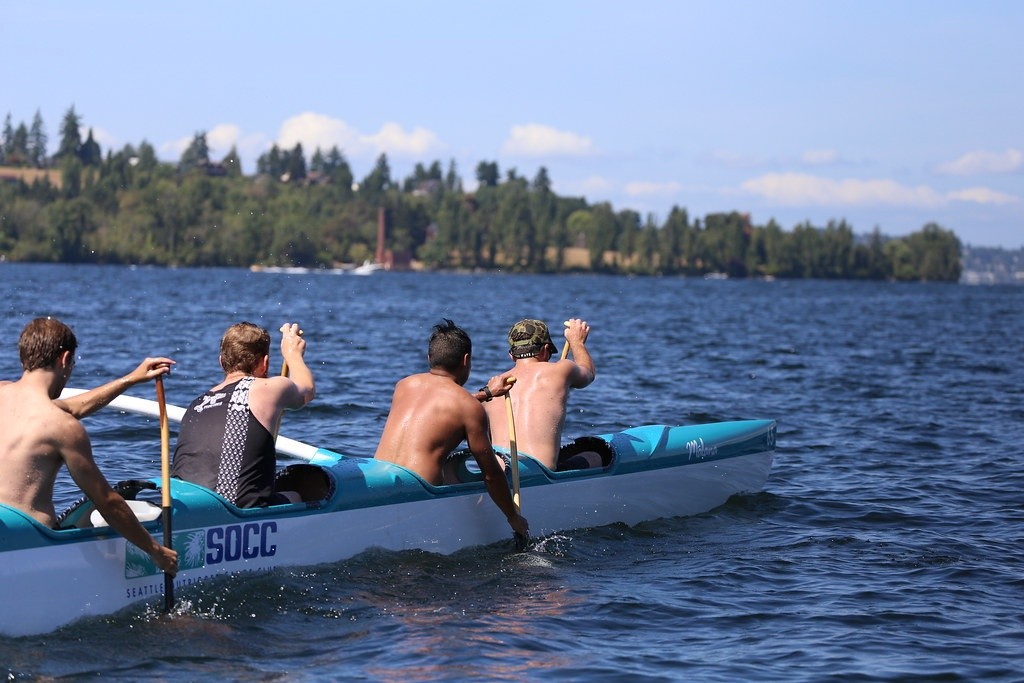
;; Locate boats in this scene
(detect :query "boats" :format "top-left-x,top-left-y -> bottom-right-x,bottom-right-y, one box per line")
351,261 -> 386,278
1,420 -> 779,638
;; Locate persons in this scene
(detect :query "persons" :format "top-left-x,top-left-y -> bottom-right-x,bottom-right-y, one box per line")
171,322 -> 316,509
481,318 -> 603,472
373,319 -> 529,535
0,316 -> 179,578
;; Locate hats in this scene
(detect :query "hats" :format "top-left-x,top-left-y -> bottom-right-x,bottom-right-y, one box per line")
508,319 -> 558,358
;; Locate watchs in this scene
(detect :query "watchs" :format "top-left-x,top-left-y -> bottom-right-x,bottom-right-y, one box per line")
478,386 -> 492,402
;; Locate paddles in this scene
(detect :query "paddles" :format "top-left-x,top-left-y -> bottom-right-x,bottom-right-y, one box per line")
271,327 -> 305,447
503,375 -> 530,551
150,362 -> 175,612
560,320 -> 584,360
